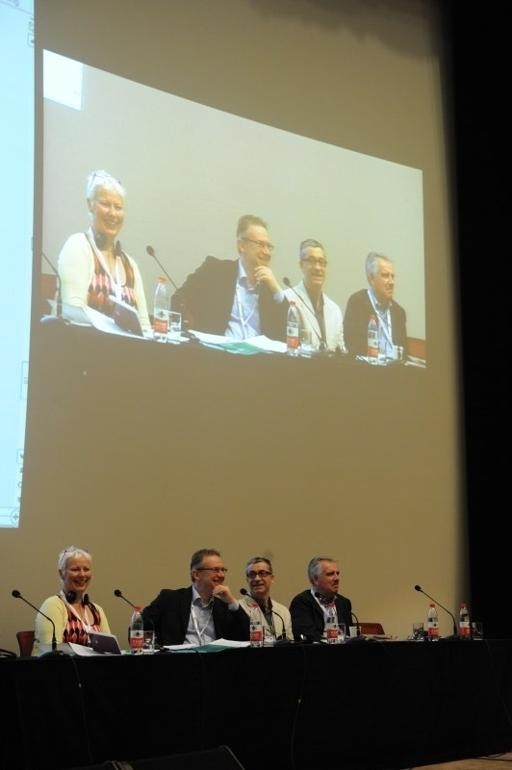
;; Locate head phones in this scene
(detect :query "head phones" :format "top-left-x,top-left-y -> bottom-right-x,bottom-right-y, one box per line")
91,227 -> 122,256
63,589 -> 89,605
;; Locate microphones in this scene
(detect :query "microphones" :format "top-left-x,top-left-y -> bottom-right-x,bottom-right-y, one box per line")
415,585 -> 474,641
240,588 -> 296,646
146,245 -> 197,343
42,252 -> 72,326
315,592 -> 367,643
114,589 -> 162,650
11,589 -> 68,658
282,277 -> 336,358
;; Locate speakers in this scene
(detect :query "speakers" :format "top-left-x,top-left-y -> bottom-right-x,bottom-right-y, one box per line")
71,745 -> 244,770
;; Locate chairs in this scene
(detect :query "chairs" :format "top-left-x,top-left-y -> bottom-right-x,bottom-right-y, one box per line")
17,631 -> 35,655
100,745 -> 244,769
407,335 -> 426,359
353,622 -> 384,635
42,274 -> 59,316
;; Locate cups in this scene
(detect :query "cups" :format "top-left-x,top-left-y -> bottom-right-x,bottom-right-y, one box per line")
472,622 -> 483,641
338,624 -> 346,644
144,631 -> 155,655
350,626 -> 361,638
413,623 -> 424,640
264,626 -> 274,647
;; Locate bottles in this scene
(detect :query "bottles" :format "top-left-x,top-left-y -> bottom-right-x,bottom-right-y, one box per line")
459,603 -> 471,641
250,603 -> 263,648
130,606 -> 144,656
427,604 -> 439,642
325,603 -> 338,644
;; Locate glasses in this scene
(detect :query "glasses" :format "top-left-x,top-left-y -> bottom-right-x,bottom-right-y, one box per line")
197,567 -> 228,574
247,570 -> 273,577
302,256 -> 329,266
241,237 -> 274,251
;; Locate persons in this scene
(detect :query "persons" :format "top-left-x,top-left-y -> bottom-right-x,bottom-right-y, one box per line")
284,236 -> 347,356
347,254 -> 411,356
233,558 -> 299,644
290,556 -> 357,646
129,549 -> 252,650
169,214 -> 295,343
52,169 -> 154,331
30,546 -> 114,659
